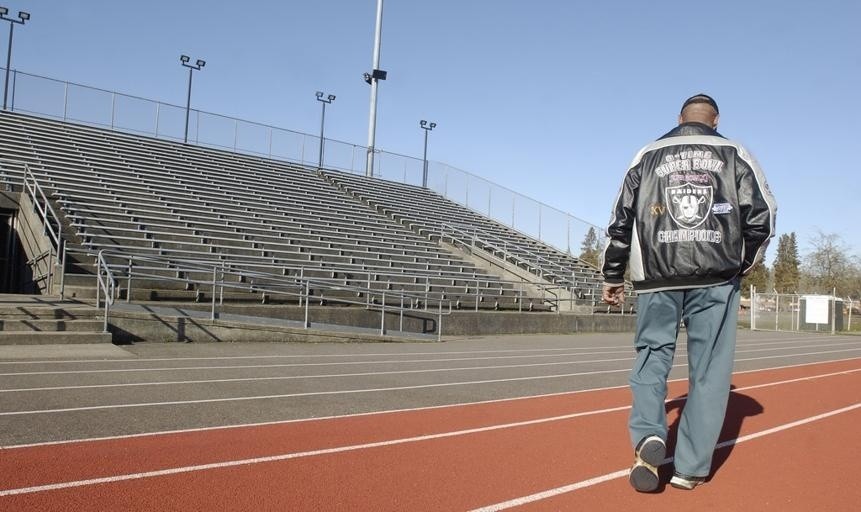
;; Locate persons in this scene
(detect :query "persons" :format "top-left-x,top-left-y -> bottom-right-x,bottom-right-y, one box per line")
602,93 -> 780,493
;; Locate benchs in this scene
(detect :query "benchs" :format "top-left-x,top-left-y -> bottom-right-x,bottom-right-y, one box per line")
2,109 -> 636,311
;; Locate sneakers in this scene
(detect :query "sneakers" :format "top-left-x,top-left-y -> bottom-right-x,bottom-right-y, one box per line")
628,434 -> 668,493
669,471 -> 706,491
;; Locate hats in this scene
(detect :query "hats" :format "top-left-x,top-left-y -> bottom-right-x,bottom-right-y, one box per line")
680,94 -> 720,114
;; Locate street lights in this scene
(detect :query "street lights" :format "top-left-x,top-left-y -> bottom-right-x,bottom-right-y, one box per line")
316,90 -> 336,170
419,119 -> 437,186
179,55 -> 206,145
0,7 -> 30,111
